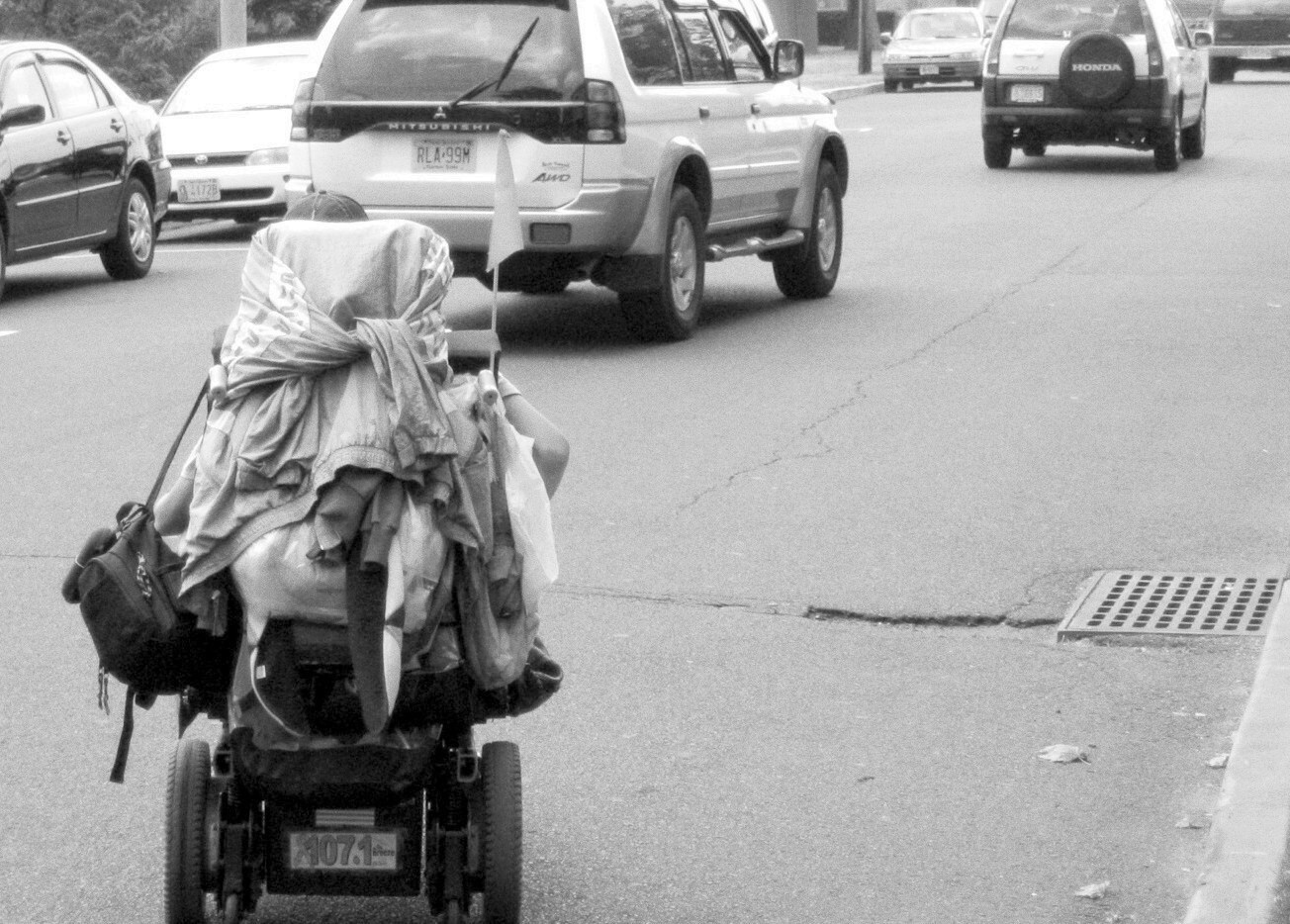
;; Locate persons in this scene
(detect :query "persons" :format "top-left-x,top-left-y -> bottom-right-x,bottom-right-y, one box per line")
153,190 -> 573,538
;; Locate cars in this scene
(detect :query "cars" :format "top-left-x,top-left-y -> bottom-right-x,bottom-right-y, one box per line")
1205,1 -> 1290,85
156,40 -> 318,218
0,39 -> 174,296
878,6 -> 994,92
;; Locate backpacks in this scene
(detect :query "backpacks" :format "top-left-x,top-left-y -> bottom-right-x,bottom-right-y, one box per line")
77,369 -> 232,711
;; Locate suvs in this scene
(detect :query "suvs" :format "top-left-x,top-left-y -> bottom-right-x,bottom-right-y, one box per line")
981,0 -> 1213,171
283,0 -> 849,343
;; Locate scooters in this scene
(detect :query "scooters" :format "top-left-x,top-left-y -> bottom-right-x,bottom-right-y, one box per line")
157,273 -> 525,924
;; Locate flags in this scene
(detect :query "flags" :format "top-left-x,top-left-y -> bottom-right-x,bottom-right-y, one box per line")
486,128 -> 524,273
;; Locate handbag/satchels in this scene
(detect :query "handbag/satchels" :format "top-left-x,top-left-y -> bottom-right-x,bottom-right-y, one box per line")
469,633 -> 562,721
